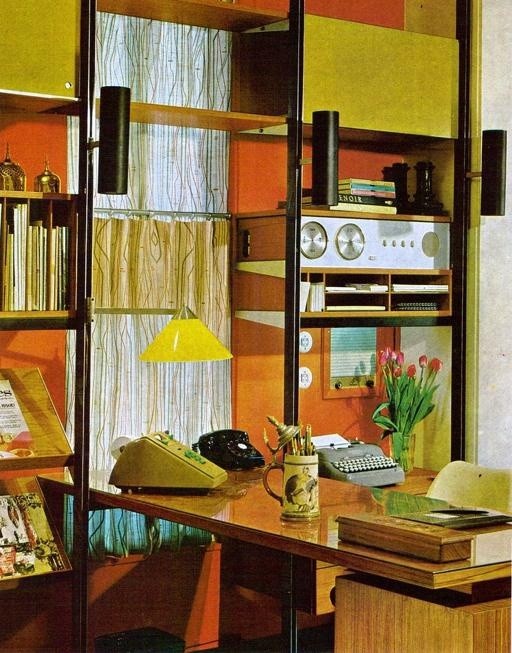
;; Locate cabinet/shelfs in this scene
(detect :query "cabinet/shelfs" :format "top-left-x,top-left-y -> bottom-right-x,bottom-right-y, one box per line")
228,1 -> 489,649
1,0 -> 95,652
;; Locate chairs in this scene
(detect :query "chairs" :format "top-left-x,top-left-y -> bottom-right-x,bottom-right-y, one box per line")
423,459 -> 512,522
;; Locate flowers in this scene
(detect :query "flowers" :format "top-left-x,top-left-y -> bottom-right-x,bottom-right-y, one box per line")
355,339 -> 446,471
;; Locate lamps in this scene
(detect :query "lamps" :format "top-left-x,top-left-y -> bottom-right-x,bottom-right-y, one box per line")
86,298 -> 237,370
466,129 -> 512,222
84,85 -> 145,195
300,108 -> 353,213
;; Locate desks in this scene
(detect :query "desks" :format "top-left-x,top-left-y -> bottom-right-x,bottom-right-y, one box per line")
28,451 -> 511,651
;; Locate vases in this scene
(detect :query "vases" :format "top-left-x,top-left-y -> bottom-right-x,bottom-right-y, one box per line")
385,431 -> 432,475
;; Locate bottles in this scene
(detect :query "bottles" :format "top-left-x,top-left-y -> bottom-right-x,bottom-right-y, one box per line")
279,513 -> 322,545
263,452 -> 320,517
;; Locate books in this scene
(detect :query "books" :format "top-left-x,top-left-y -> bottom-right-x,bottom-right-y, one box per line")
302,177 -> 397,216
0,199 -> 70,310
0,364 -> 74,466
0,475 -> 74,580
300,281 -> 449,312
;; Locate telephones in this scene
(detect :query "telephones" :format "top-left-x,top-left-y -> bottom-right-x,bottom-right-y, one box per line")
191,429 -> 265,472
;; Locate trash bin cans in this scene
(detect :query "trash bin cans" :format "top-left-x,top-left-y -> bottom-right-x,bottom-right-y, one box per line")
94,625 -> 185,652
